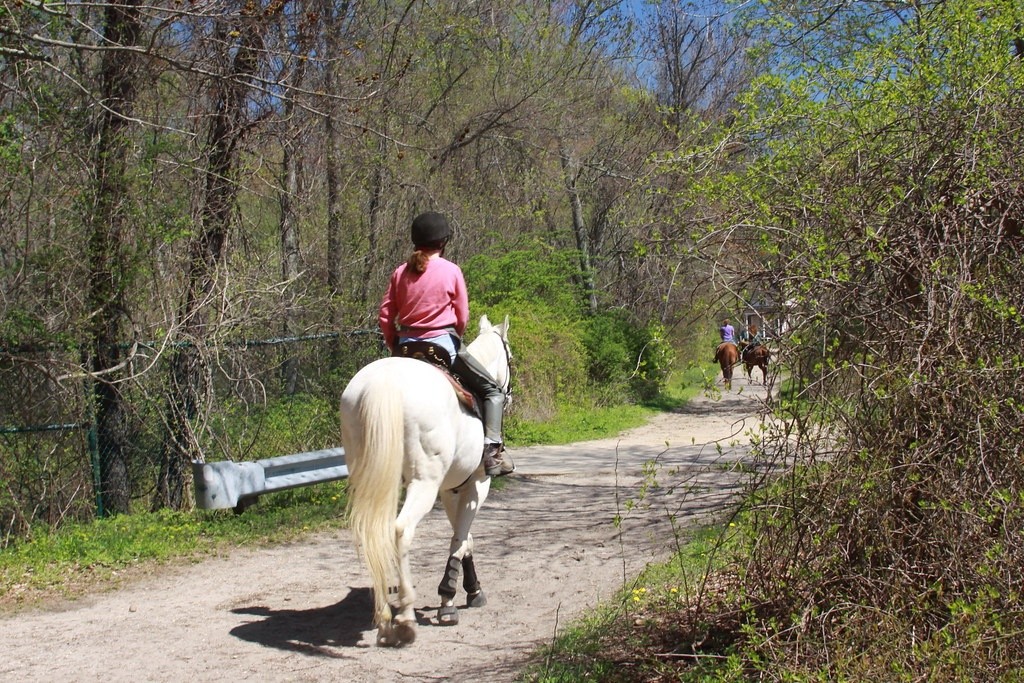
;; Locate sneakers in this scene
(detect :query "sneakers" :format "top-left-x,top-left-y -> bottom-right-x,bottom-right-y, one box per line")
483,444 -> 515,475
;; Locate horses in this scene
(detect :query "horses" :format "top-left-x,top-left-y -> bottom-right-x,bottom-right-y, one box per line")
340,313 -> 515,647
716,339 -> 741,390
739,340 -> 771,387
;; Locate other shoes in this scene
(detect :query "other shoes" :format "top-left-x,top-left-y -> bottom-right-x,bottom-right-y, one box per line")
738,359 -> 743,362
712,358 -> 717,363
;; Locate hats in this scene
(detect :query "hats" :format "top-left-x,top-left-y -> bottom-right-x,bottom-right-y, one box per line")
723,319 -> 730,324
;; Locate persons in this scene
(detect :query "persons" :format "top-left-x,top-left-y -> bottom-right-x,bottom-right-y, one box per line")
712,319 -> 741,363
378,212 -> 514,475
741,324 -> 763,363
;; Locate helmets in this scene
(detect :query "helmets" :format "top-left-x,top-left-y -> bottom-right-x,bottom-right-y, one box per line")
411,212 -> 450,241
